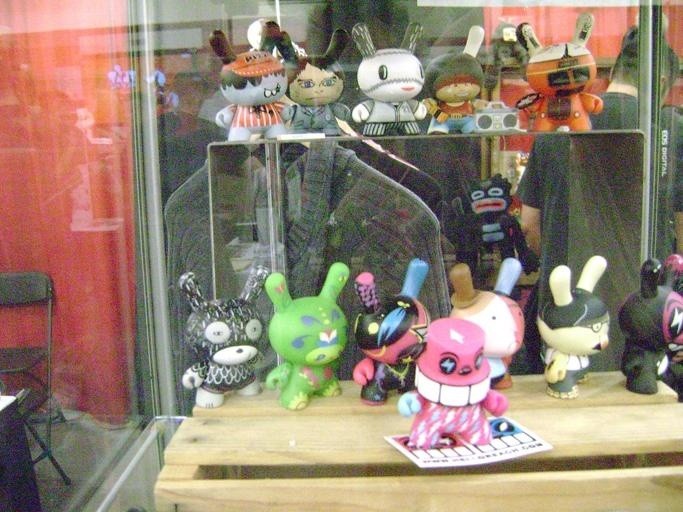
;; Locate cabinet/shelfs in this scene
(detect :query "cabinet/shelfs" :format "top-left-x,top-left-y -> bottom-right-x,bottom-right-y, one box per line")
482,5 -> 683,304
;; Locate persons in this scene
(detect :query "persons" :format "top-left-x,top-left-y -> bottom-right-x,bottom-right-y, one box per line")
516,27 -> 683,262
306,0 -> 480,278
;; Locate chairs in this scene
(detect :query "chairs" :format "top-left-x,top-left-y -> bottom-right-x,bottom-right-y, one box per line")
0,272 -> 70,487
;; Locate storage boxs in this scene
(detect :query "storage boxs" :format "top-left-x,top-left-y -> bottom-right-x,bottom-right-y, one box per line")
153,371 -> 683,512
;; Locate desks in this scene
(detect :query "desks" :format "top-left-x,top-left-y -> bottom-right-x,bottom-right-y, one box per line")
0,395 -> 44,512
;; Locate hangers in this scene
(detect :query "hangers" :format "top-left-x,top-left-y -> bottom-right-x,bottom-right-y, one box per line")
285,113 -> 364,149
